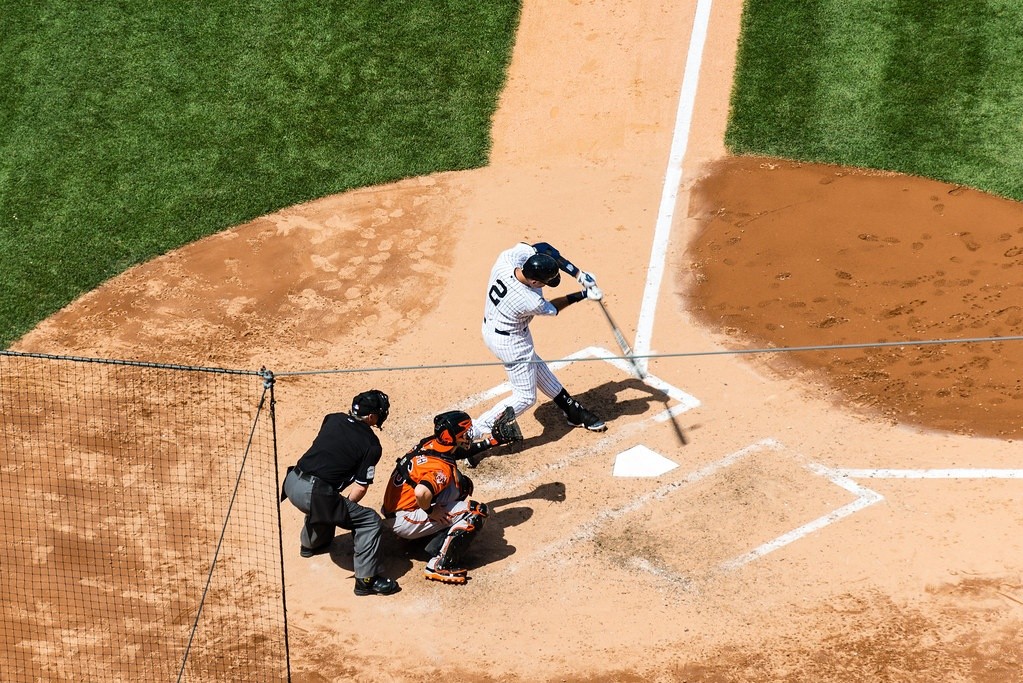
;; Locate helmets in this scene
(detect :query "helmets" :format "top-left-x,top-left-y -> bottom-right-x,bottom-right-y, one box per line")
434,410 -> 474,451
352,389 -> 390,431
523,254 -> 561,287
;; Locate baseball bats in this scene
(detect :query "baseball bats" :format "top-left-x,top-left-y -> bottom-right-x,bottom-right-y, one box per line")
598,300 -> 646,382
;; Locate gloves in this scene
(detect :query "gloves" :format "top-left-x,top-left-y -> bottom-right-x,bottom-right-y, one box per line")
577,270 -> 596,288
587,286 -> 602,300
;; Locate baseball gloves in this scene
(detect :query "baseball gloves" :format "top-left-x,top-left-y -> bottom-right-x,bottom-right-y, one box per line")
492,406 -> 525,446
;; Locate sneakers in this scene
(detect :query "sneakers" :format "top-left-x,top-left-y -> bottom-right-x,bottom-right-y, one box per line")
300,531 -> 333,557
354,574 -> 399,595
566,400 -> 606,431
424,557 -> 465,584
463,456 -> 475,468
392,533 -> 413,544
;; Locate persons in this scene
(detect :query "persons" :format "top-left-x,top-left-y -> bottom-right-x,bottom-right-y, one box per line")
465,241 -> 607,469
381,410 -> 490,585
280,389 -> 402,596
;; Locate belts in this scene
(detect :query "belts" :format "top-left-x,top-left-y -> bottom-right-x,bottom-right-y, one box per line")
293,466 -> 317,484
484,317 -> 527,336
384,512 -> 396,519
